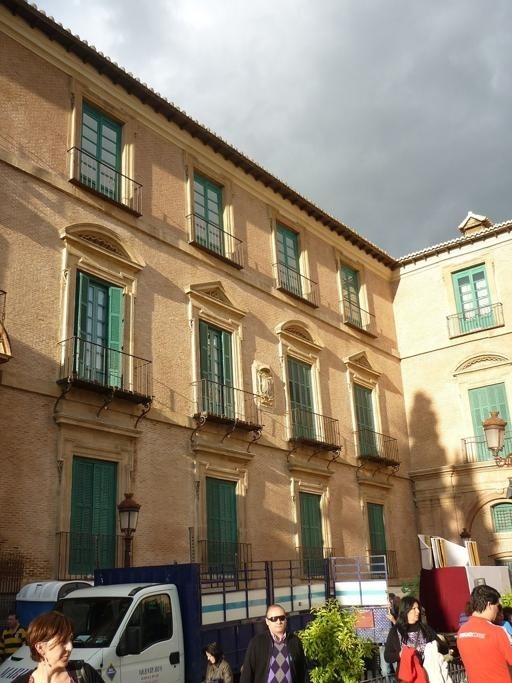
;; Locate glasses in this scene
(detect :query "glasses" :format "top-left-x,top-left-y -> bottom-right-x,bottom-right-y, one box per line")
265,615 -> 286,622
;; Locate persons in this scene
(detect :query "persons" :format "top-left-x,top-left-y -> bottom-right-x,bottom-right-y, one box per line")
0,612 -> 28,657
239,604 -> 309,683
10,612 -> 105,683
384,585 -> 512,683
204,640 -> 234,683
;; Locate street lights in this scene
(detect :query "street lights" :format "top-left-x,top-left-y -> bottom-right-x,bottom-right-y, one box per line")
116,490 -> 142,568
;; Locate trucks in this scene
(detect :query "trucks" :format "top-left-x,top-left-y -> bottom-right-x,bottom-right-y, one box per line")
0,554 -> 392,682
